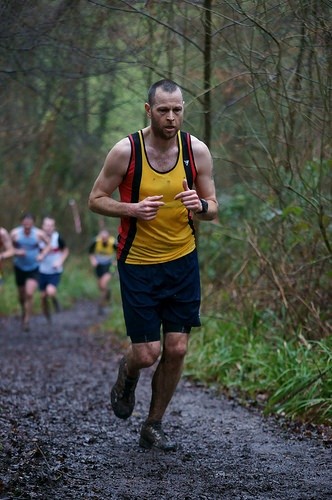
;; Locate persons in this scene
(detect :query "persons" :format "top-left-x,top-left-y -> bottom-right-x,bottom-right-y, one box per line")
88,228 -> 119,315
88,79 -> 219,452
10,212 -> 52,333
37,214 -> 70,325
0,226 -> 16,286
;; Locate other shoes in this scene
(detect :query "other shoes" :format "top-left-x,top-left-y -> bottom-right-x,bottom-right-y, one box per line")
139,422 -> 176,450
111,353 -> 141,419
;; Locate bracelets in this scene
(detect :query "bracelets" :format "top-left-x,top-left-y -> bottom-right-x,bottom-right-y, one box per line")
195,198 -> 208,214
